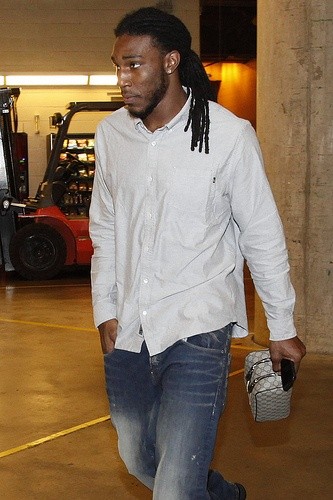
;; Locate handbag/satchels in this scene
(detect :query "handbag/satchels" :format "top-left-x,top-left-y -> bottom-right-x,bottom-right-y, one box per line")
244,349 -> 293,423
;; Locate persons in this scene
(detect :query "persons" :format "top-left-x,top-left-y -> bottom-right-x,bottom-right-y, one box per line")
89,5 -> 307,500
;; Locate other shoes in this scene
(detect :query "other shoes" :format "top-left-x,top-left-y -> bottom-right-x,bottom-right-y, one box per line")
233,481 -> 247,500
